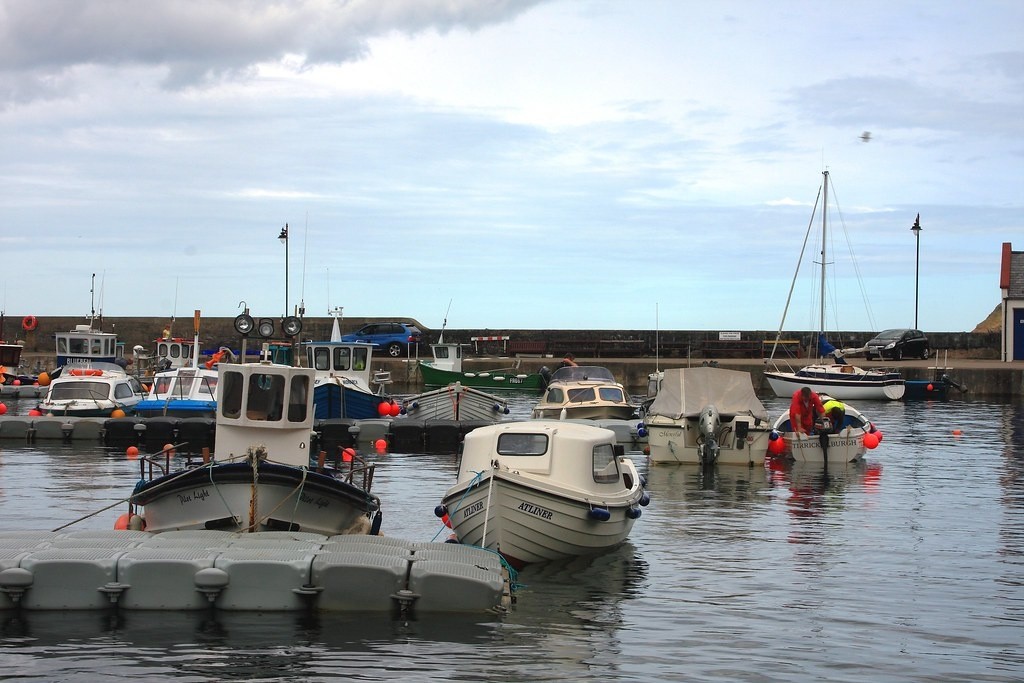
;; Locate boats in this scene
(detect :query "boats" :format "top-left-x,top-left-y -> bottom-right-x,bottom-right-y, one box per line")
37,368 -> 149,420
903,348 -> 968,402
400,381 -> 511,421
770,391 -> 882,463
638,364 -> 774,467
638,369 -> 667,419
0,311 -> 38,385
431,417 -> 652,570
50,273 -> 131,380
115,341 -> 382,537
297,304 -> 400,420
413,323 -> 545,395
531,366 -> 640,422
129,308 -> 219,418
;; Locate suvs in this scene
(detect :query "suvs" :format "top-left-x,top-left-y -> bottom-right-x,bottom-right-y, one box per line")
862,329 -> 931,361
340,321 -> 424,358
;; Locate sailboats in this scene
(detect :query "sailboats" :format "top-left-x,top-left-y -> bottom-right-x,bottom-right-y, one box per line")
764,165 -> 907,400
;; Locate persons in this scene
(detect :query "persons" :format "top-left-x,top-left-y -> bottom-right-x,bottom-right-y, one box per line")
789,387 -> 825,435
554,353 -> 578,403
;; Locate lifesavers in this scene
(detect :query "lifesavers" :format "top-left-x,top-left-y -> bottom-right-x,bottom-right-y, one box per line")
272,341 -> 292,347
69,368 -> 103,378
22,314 -> 38,332
